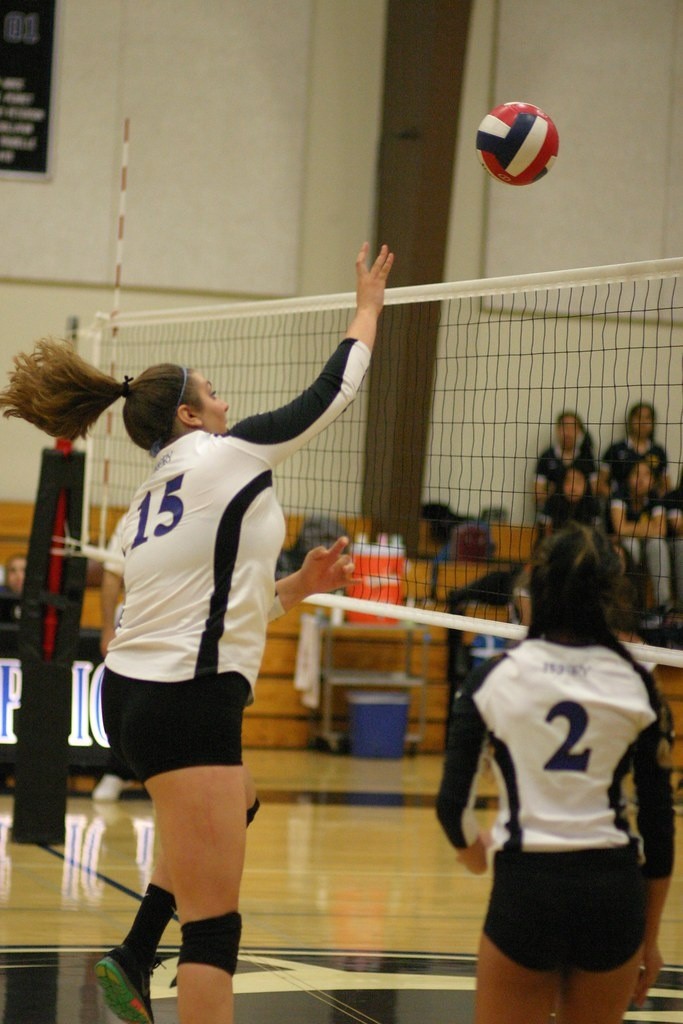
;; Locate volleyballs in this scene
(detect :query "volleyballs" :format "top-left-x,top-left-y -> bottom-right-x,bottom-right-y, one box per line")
475,102 -> 559,186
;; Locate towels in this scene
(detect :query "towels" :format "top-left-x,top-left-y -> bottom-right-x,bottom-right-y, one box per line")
292,612 -> 322,709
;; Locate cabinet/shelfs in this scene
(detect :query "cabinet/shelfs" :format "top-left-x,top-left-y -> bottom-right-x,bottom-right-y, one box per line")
323,610 -> 427,754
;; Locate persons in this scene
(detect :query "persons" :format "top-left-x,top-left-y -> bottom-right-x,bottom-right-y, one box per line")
435,524 -> 675,1024
0,553 -> 29,623
512,403 -> 683,667
0,240 -> 394,1024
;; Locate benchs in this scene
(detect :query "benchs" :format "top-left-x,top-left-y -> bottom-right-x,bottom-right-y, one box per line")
0,500 -> 683,773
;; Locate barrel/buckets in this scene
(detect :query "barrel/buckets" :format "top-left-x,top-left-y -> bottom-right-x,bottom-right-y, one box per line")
346,544 -> 407,624
348,691 -> 407,759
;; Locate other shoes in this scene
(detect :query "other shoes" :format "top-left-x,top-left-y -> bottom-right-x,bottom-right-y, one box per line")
93,774 -> 134,801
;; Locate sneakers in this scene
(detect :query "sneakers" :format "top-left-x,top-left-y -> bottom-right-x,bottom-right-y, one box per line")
94,944 -> 167,1024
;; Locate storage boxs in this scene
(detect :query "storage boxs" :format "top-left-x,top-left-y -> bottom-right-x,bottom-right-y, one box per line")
343,692 -> 408,758
